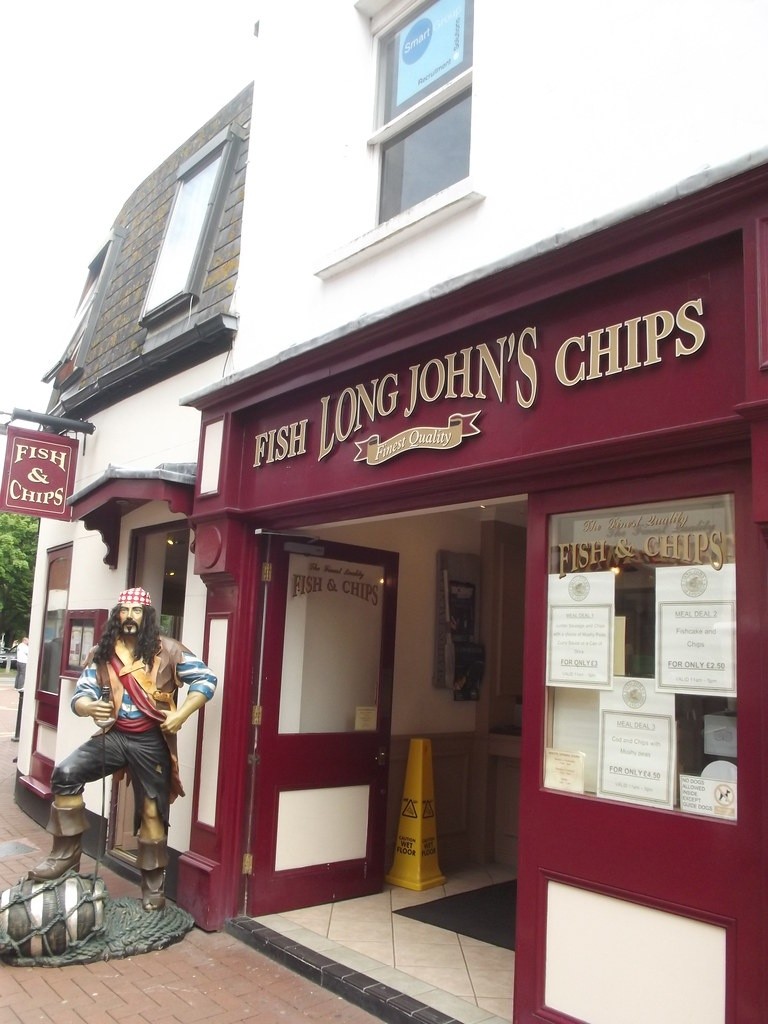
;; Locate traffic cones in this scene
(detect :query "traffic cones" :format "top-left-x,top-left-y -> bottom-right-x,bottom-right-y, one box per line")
384,738 -> 448,892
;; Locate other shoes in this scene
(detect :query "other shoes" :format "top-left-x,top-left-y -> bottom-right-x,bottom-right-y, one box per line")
15,687 -> 24,691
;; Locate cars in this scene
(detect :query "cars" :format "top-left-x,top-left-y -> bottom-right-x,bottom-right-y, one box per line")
0,644 -> 18,669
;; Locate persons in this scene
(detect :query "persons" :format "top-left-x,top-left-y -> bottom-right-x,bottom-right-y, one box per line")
14,637 -> 28,691
26,587 -> 217,912
